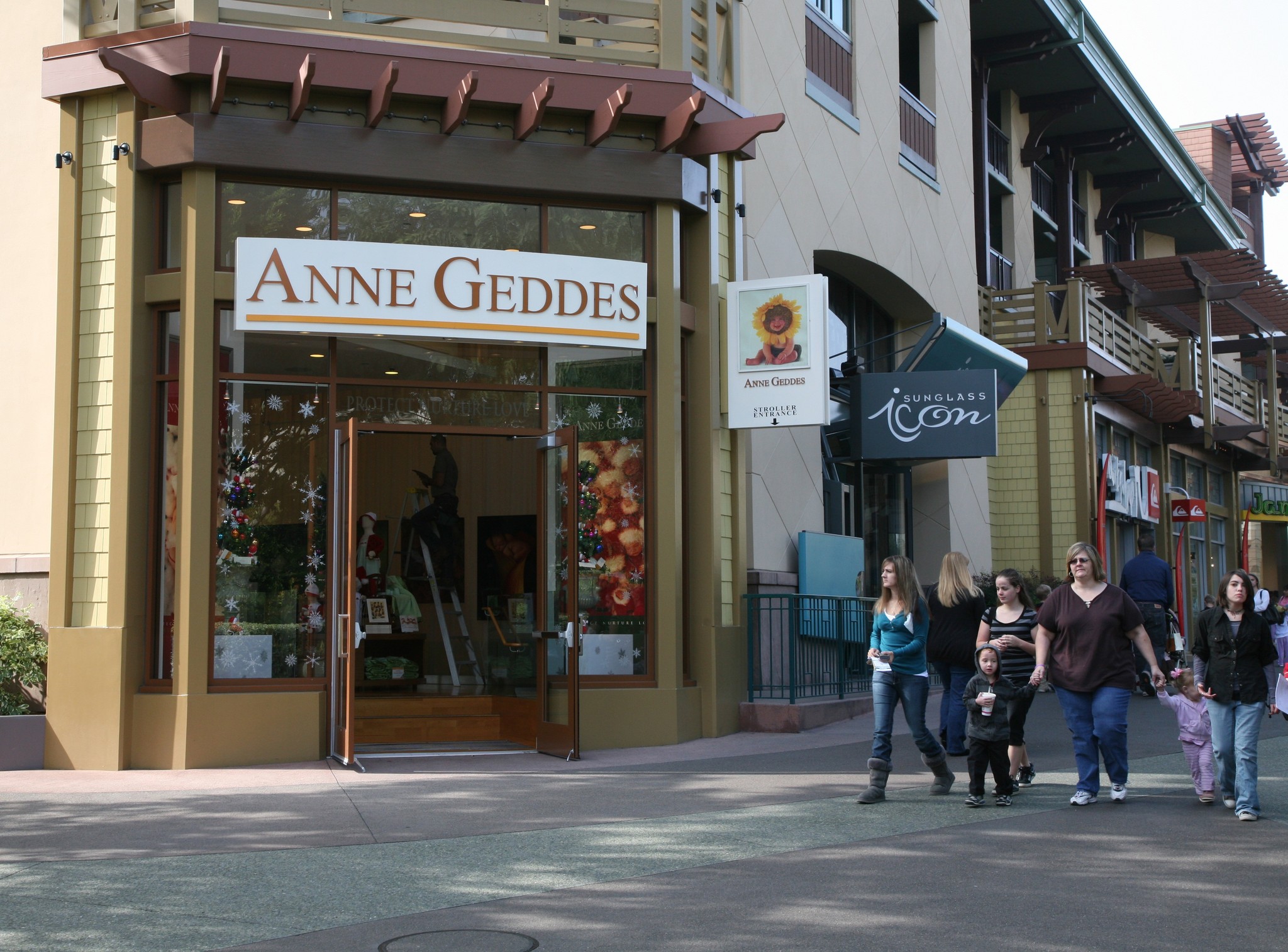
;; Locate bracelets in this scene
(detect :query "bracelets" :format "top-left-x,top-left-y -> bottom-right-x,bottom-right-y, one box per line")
1034,664 -> 1045,670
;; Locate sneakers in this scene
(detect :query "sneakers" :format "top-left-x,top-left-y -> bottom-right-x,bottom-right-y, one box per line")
1070,790 -> 1097,805
1018,762 -> 1036,787
1222,794 -> 1236,808
964,794 -> 985,805
1110,780 -> 1130,801
995,794 -> 1013,806
1238,810 -> 1257,820
991,775 -> 1019,795
1141,671 -> 1157,696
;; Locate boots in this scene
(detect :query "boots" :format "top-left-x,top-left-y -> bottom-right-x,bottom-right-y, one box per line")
921,746 -> 955,795
857,758 -> 892,803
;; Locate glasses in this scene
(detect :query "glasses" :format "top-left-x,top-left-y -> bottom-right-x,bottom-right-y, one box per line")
1069,558 -> 1091,564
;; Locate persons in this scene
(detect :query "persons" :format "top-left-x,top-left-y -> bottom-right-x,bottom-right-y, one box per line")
961,643 -> 1040,806
1202,573 -> 1288,667
409,435 -> 459,588
1033,585 -> 1056,693
355,512 -> 383,598
1028,542 -> 1164,806
1119,534 -> 1175,695
1190,570 -> 1279,821
924,552 -> 987,757
1155,668 -> 1215,802
1282,663 -> 1288,721
301,584 -> 324,620
854,555 -> 957,803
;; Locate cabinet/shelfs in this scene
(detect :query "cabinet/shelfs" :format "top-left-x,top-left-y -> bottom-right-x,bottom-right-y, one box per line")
354,629 -> 428,693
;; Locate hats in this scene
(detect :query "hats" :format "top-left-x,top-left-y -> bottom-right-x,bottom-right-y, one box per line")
1274,603 -> 1284,612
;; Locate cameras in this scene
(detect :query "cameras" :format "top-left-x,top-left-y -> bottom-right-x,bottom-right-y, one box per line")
879,653 -> 890,660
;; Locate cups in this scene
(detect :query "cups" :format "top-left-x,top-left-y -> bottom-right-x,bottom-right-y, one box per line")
981,692 -> 996,716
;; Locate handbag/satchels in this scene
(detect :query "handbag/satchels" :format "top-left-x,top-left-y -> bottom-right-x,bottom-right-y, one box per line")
1260,590 -> 1281,625
1166,622 -> 1184,652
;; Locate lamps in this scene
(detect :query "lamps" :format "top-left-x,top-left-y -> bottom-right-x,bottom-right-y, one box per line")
112,142 -> 130,160
1163,432 -> 1220,455
56,151 -> 73,168
736,202 -> 745,218
1085,389 -> 1154,419
1250,177 -> 1259,193
1233,461 -> 1283,480
712,188 -> 721,204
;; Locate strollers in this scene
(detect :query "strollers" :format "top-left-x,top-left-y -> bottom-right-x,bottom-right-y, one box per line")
1164,608 -> 1190,686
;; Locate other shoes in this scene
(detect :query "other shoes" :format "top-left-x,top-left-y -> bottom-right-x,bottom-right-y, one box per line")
947,748 -> 970,756
1047,683 -> 1055,692
1202,791 -> 1216,800
1039,689 -> 1049,692
1199,794 -> 1214,802
